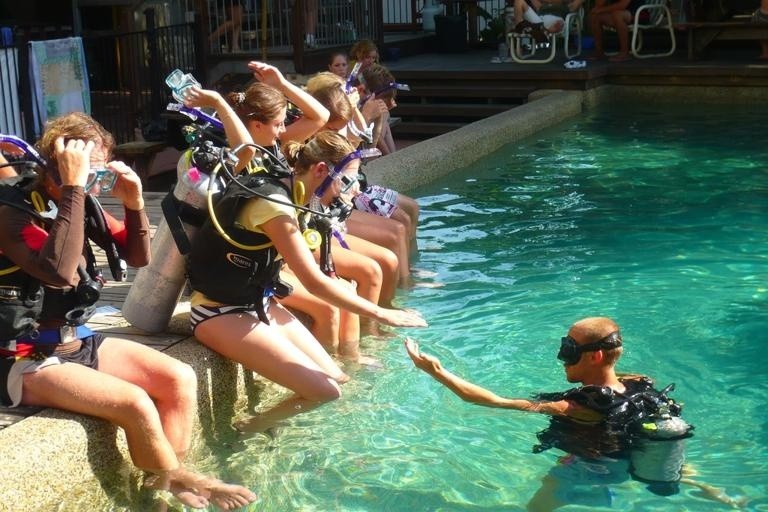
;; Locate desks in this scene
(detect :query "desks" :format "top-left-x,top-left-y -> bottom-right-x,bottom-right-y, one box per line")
686,19 -> 768,60
110,141 -> 164,190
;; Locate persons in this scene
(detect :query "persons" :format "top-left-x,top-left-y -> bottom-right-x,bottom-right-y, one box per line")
0,110 -> 261,510
0,152 -> 19,180
292,0 -> 320,49
329,41 -> 398,156
209,1 -> 246,53
588,0 -> 652,62
182,61 -> 431,400
505,0 -> 584,33
406,316 -> 745,512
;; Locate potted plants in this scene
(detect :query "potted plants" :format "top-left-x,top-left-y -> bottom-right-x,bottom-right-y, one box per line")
433,0 -> 490,55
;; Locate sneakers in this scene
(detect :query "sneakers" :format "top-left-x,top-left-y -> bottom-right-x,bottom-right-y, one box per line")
302,33 -> 319,51
490,54 -> 518,65
562,58 -> 588,70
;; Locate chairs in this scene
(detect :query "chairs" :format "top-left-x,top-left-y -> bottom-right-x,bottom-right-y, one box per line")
508,0 -> 676,65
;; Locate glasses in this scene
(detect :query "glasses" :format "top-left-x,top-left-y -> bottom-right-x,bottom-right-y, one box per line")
84,169 -> 118,194
164,68 -> 201,104
324,162 -> 366,195
556,336 -> 584,367
